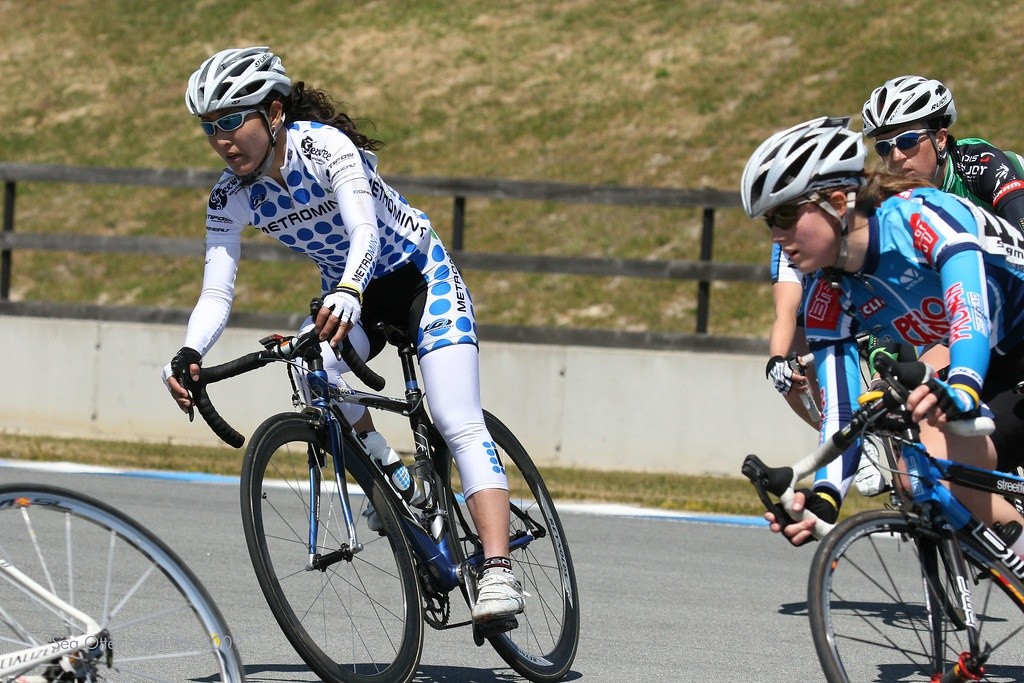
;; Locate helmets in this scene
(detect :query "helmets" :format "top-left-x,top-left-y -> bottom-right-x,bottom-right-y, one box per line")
741,116 -> 868,220
861,75 -> 957,138
185,46 -> 294,115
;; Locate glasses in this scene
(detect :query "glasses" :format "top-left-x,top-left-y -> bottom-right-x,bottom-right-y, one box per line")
762,200 -> 810,230
200,108 -> 259,136
872,129 -> 938,156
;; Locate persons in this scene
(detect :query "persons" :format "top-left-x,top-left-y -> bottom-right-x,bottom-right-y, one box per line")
862,75 -> 1024,236
161,47 -> 526,619
739,117 -> 1024,561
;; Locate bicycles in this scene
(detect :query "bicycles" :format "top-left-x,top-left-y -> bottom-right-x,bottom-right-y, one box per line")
777,326 -> 999,628
0,477 -> 244,683
740,356 -> 1024,683
169,297 -> 584,683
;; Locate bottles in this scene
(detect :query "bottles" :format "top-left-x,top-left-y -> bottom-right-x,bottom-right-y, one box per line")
992,520 -> 1024,562
359,430 -> 419,504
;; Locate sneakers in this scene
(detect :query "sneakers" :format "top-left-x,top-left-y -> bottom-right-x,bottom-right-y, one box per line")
363,502 -> 383,531
472,566 -> 531,621
